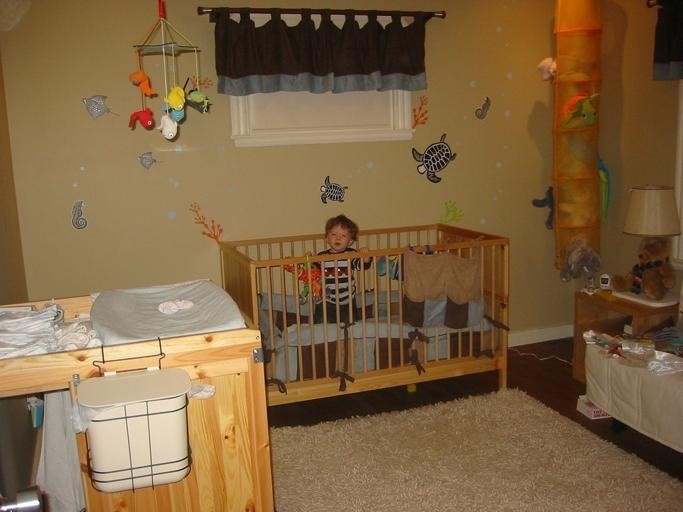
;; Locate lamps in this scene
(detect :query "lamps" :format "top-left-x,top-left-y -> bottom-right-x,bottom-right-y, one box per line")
622,186 -> 681,238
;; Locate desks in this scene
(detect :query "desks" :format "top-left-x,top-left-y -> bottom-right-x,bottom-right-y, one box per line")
0,279 -> 274,511
572,288 -> 680,381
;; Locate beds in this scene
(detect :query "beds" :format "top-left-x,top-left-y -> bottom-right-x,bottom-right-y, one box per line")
219,224 -> 509,406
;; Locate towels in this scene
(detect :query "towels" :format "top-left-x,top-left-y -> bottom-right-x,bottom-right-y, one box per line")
28,390 -> 84,511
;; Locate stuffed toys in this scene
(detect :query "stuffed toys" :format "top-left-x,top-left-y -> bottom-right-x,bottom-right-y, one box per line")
286,255 -> 322,305
611,237 -> 676,301
531,55 -> 602,283
187,88 -> 209,113
157,86 -> 186,140
128,69 -> 157,96
129,108 -> 153,130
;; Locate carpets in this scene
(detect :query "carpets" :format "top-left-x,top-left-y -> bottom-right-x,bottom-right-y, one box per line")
270,386 -> 682,511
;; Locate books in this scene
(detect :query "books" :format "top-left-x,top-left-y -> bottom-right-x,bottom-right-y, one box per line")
576,394 -> 611,420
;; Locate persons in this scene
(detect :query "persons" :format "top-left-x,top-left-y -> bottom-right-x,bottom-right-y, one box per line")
301,214 -> 370,325
